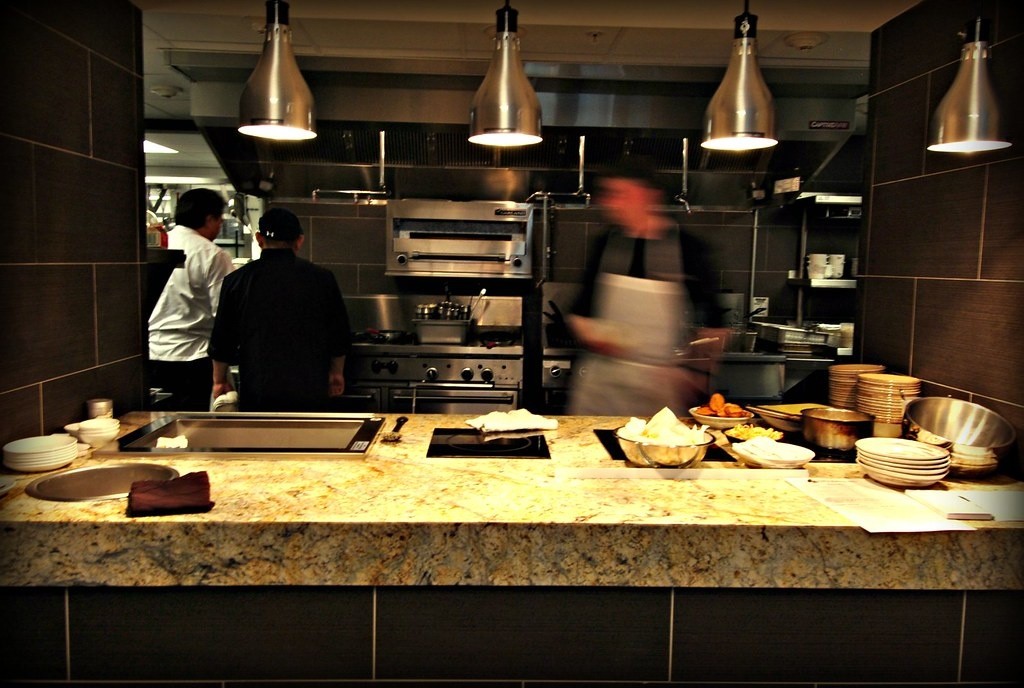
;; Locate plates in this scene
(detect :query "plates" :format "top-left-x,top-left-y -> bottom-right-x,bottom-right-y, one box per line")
0,477 -> 16,497
854,437 -> 949,486
690,405 -> 815,470
3,435 -> 80,470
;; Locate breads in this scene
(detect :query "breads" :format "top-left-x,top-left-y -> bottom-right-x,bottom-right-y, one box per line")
693,392 -> 750,418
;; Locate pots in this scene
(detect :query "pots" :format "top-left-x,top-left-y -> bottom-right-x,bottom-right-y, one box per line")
743,404 -> 875,451
479,332 -> 515,348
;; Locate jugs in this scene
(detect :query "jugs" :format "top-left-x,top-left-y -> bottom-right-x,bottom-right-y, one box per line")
147,210 -> 175,248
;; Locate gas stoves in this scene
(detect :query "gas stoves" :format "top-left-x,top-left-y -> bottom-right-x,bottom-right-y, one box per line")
346,339 -> 523,390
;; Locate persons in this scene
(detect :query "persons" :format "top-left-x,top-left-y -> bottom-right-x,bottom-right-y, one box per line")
148,188 -> 237,412
555,163 -> 733,416
207,208 -> 350,413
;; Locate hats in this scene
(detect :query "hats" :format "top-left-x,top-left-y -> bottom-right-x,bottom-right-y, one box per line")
260,209 -> 304,242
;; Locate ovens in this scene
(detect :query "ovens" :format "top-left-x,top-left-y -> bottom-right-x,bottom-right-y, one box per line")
333,383 -> 518,414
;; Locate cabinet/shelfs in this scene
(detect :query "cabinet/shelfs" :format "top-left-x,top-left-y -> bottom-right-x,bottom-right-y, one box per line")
780,194 -> 863,289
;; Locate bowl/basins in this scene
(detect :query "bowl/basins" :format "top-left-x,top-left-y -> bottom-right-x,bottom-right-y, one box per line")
902,396 -> 1016,479
757,364 -> 922,438
613,424 -> 716,470
366,326 -> 405,339
64,418 -> 123,448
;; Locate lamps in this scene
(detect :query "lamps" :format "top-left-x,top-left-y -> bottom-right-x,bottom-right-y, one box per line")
467,0 -> 544,147
927,0 -> 1013,155
700,0 -> 780,152
234,0 -> 317,143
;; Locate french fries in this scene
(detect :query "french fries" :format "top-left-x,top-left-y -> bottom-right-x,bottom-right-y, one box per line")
724,424 -> 783,440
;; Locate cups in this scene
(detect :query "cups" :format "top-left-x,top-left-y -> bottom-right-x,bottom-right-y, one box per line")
89,398 -> 113,418
807,250 -> 845,281
840,324 -> 854,347
